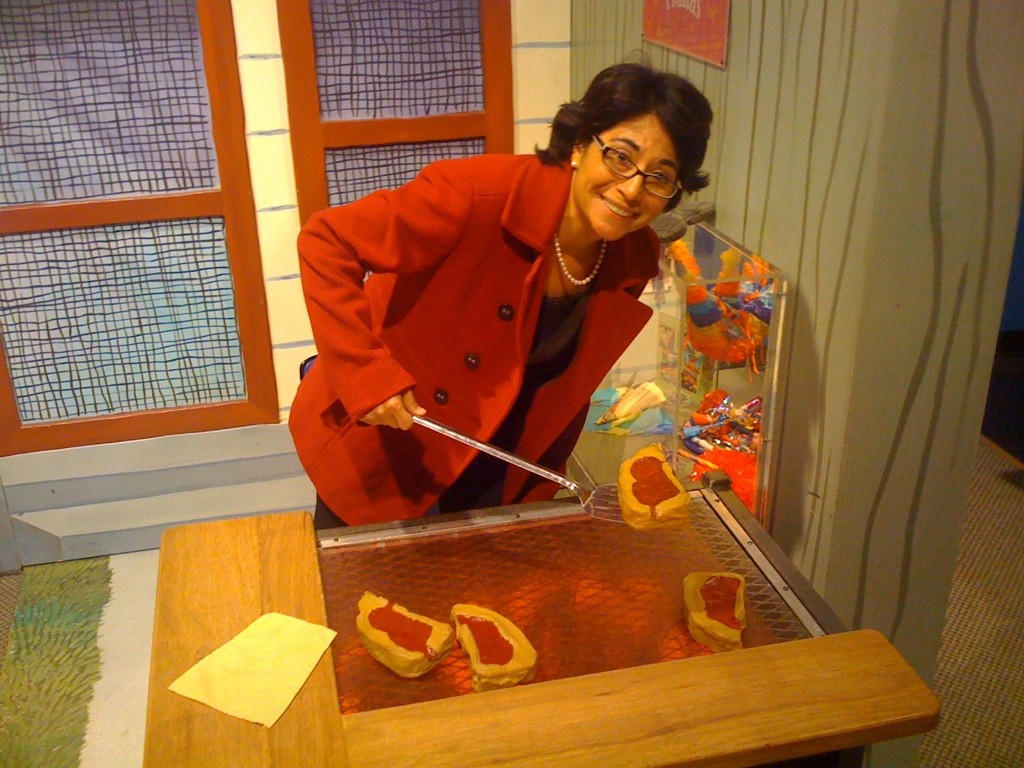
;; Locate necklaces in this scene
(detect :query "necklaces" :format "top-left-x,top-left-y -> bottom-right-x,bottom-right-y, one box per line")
554,235 -> 608,286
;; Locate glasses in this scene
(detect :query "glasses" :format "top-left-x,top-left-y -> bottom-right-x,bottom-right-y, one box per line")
594,132 -> 683,200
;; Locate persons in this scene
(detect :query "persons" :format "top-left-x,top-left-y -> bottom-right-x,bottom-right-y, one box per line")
288,49 -> 714,531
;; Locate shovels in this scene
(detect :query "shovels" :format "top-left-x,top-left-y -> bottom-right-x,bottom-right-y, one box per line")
411,414 -> 626,524
